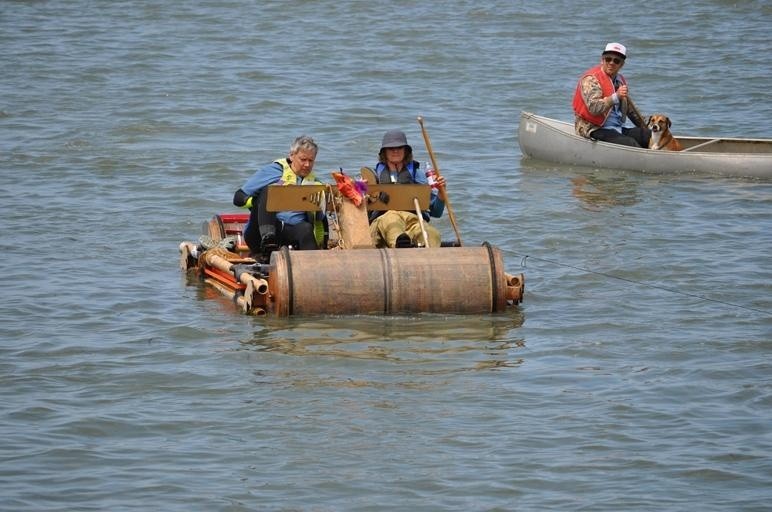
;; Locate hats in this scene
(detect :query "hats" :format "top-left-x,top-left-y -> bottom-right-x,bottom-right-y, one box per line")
380,130 -> 412,155
602,43 -> 627,59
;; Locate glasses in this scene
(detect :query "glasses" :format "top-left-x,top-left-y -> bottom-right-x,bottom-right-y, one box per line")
604,56 -> 620,64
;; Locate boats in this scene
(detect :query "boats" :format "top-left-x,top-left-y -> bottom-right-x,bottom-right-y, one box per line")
178,183 -> 525,318
518,111 -> 772,178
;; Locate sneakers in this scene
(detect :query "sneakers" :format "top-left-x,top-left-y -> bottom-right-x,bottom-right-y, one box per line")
260,234 -> 280,257
396,232 -> 411,248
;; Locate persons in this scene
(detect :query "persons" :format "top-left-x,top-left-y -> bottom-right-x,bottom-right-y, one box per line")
362,129 -> 447,249
233,135 -> 329,275
572,42 -> 651,149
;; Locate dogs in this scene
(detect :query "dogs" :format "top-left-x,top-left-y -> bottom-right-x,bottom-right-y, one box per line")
647,114 -> 685,152
